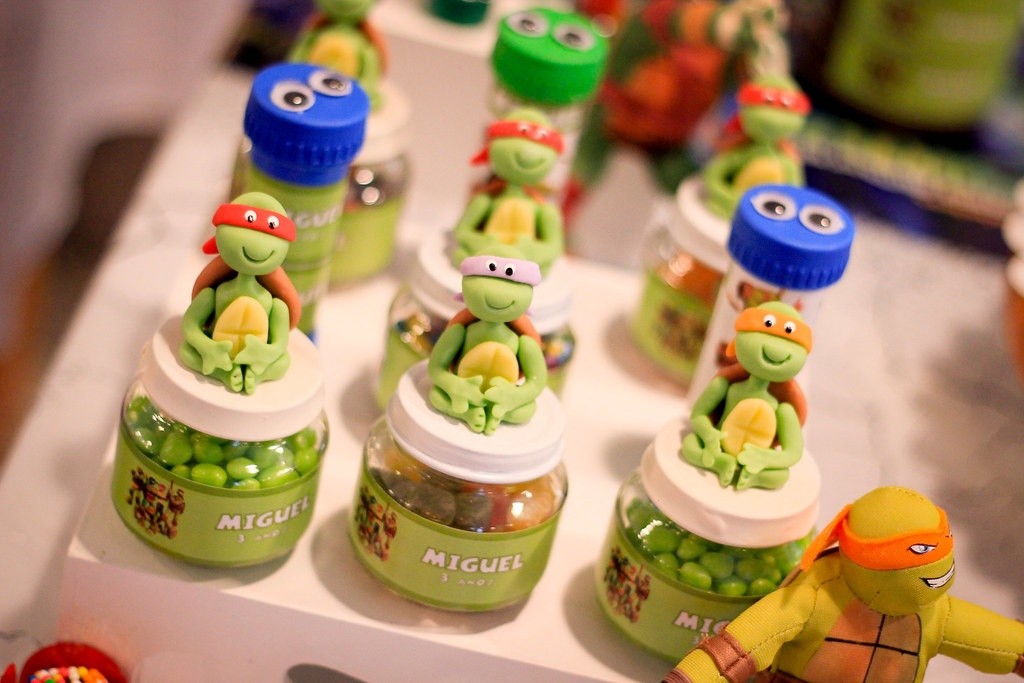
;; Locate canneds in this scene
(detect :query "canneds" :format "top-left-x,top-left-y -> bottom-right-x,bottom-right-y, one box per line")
109,79 -> 848,656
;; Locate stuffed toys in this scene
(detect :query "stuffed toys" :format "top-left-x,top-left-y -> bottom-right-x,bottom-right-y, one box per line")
660,488 -> 1024,683
178,0 -> 810,490
